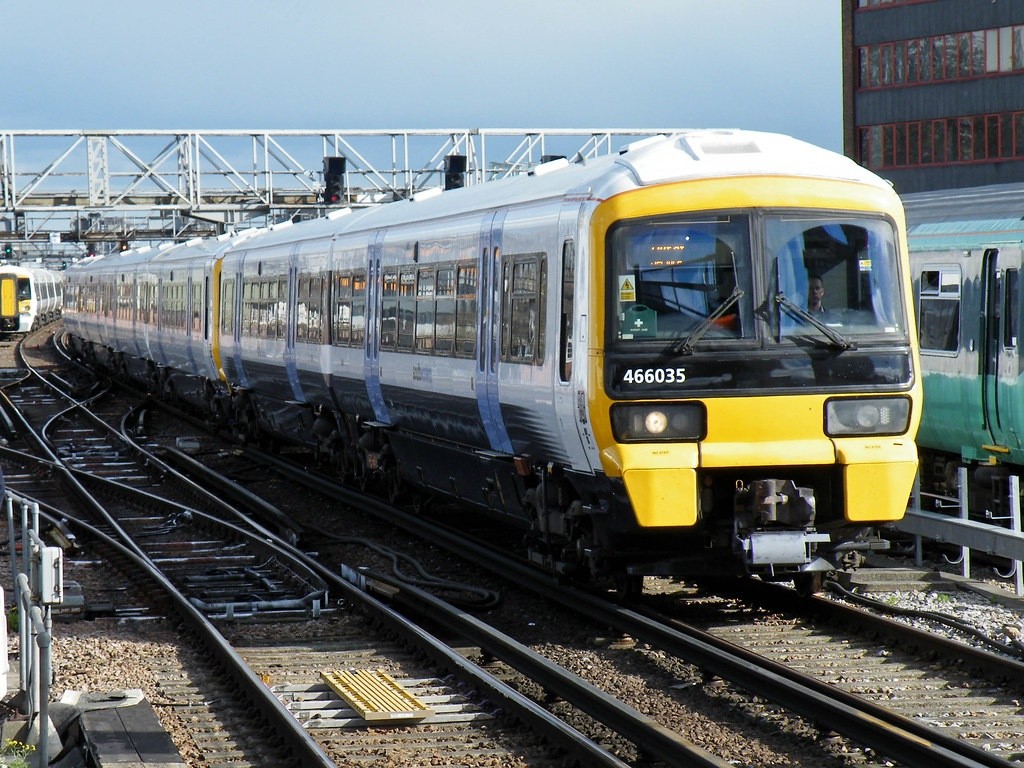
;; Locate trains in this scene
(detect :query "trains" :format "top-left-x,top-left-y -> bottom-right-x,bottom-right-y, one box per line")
61,128 -> 923,610
0,262 -> 61,337
898,182 -> 1022,565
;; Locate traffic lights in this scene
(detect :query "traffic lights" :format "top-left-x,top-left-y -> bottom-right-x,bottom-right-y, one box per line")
323,157 -> 346,205
120,242 -> 127,252
63,262 -> 66,269
444,155 -> 467,190
5,245 -> 12,260
290,211 -> 302,223
88,244 -> 95,257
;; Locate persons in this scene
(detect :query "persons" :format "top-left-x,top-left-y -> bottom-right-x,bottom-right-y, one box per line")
795,273 -> 852,328
